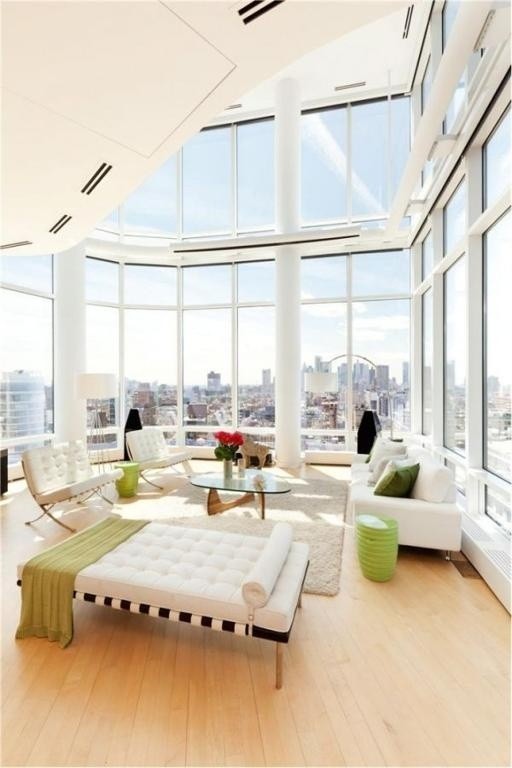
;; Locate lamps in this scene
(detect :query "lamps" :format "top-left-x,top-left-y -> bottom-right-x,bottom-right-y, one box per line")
169,220 -> 364,261
302,353 -> 395,438
79,374 -> 119,494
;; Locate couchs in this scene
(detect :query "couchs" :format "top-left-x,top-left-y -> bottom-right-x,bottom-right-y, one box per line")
350,440 -> 462,562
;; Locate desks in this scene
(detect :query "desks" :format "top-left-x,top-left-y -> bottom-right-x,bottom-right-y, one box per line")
0,447 -> 9,495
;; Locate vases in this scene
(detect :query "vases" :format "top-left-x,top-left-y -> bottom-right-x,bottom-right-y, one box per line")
224,460 -> 233,477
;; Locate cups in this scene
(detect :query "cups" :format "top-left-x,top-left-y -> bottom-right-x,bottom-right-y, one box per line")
237,457 -> 246,472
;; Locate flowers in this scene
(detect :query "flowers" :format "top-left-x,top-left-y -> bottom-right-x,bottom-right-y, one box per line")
213,430 -> 244,462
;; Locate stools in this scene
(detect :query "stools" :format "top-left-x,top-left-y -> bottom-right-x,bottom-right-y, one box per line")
114,461 -> 142,498
356,516 -> 401,582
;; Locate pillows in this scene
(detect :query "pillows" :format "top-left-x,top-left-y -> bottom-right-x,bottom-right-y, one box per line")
240,523 -> 292,607
365,436 -> 420,498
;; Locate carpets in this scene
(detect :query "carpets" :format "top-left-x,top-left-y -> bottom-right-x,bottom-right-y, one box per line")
154,478 -> 349,597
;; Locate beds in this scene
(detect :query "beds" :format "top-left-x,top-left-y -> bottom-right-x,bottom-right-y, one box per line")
16,514 -> 311,691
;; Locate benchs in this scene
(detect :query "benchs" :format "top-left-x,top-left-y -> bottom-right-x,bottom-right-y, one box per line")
22,440 -> 123,531
125,427 -> 191,489
